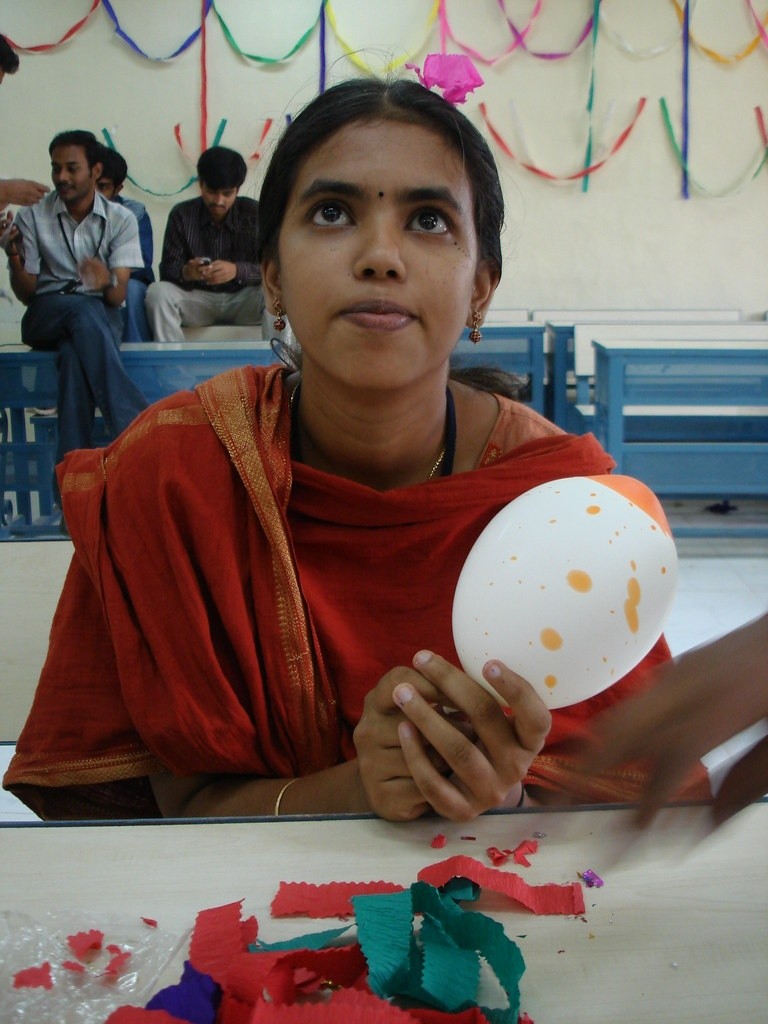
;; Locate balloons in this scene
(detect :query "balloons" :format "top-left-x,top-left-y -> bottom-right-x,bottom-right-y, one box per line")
451,474 -> 679,715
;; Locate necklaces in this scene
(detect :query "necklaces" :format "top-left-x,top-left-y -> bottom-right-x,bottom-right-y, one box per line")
290,376 -> 446,481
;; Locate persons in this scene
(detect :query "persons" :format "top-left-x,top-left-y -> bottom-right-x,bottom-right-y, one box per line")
588,608 -> 768,828
0,35 -> 261,536
3,76 -> 715,823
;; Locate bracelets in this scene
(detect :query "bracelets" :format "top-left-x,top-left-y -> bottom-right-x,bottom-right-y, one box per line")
5,249 -> 17,257
517,784 -> 524,807
274,778 -> 302,817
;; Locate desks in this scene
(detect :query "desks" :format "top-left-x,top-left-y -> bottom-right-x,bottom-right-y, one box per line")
0,312 -> 768,542
0,798 -> 768,1024
